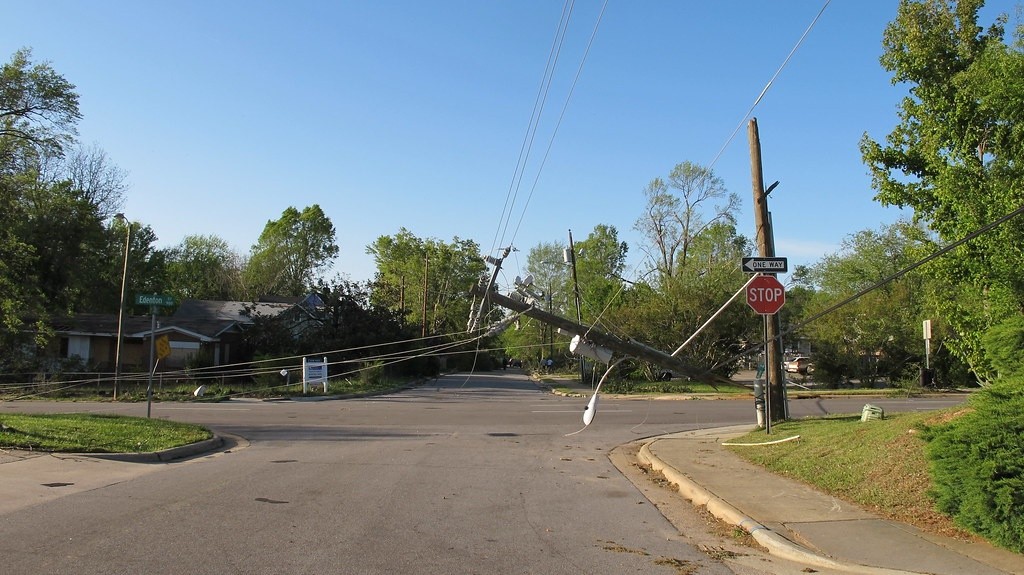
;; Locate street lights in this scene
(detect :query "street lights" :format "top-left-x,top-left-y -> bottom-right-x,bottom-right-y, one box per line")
113,213 -> 132,401
540,259 -> 586,383
582,346 -> 766,426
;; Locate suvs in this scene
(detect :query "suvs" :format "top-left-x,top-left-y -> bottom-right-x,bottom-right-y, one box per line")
788,357 -> 812,375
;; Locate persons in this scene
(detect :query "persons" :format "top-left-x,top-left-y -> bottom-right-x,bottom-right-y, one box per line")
502,358 -> 507,370
540,358 -> 554,369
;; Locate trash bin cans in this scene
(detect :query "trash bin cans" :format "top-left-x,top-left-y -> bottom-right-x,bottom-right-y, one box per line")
753,378 -> 766,427
919,368 -> 937,388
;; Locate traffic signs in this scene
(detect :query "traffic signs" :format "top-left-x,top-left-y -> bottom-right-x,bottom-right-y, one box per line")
136,293 -> 175,306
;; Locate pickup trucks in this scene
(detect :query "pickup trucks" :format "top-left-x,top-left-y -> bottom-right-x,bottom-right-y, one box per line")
782,359 -> 802,371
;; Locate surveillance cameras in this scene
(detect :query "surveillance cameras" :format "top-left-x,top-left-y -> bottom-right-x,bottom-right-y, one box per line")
518,275 -> 533,291
578,248 -> 584,256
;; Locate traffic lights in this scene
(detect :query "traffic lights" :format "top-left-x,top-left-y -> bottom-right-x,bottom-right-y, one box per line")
741,257 -> 788,273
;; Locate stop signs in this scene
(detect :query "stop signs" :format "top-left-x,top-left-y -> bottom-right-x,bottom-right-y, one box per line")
746,275 -> 785,314
